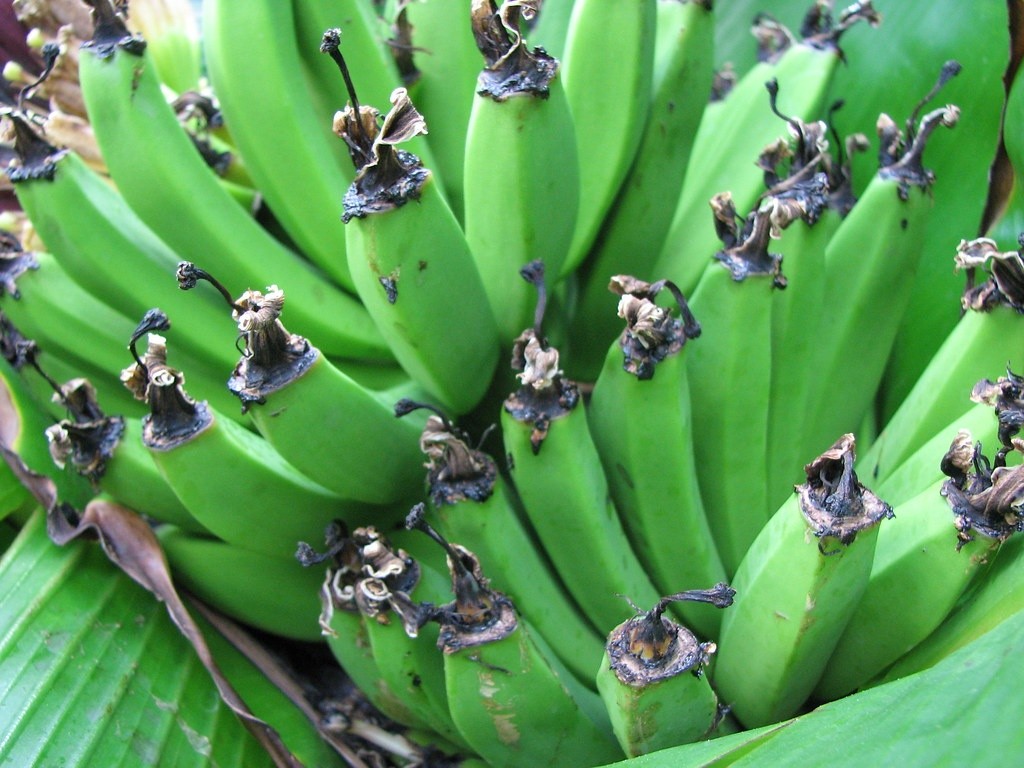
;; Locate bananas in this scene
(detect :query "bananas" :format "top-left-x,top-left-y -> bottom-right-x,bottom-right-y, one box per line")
0,0 -> 1024,768
